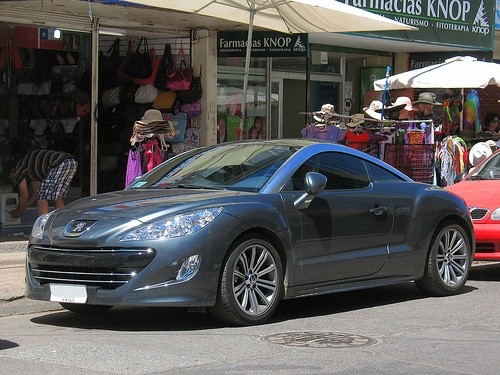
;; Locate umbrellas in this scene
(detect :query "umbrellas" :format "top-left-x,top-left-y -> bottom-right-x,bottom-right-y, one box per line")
119,0 -> 419,142
374,56 -> 500,131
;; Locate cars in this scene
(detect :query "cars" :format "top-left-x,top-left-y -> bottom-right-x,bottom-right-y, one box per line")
23,139 -> 478,328
441,147 -> 500,279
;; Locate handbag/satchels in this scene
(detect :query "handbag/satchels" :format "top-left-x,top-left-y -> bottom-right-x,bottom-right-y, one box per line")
71,111 -> 200,154
83,38 -> 202,125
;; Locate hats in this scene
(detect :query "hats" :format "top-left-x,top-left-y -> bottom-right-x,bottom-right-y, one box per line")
375,103 -> 406,119
135,109 -> 168,137
469,142 -> 493,167
412,93 -> 443,106
345,113 -> 366,128
391,96 -> 413,111
312,104 -> 340,124
362,99 -> 385,120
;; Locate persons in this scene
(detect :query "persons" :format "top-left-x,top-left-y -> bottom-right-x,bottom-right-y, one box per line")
482,112 -> 500,133
249,117 -> 266,139
217,119 -> 227,144
2,149 -> 79,219
